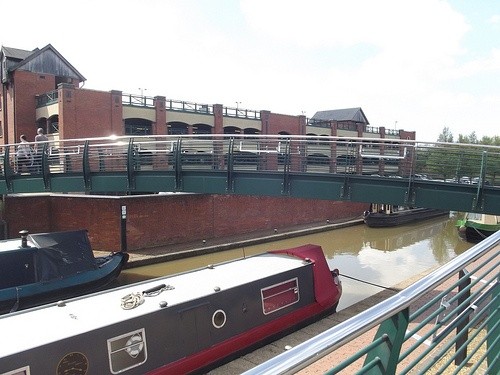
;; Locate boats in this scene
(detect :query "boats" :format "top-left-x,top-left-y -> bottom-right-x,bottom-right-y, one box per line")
456,211 -> 500,243
0,228 -> 130,316
363,202 -> 451,228
0,243 -> 342,375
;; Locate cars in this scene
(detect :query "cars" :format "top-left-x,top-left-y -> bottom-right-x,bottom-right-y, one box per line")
369,172 -> 489,187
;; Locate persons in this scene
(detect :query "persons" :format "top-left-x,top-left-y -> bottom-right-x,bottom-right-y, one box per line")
33,128 -> 50,174
15,134 -> 36,176
